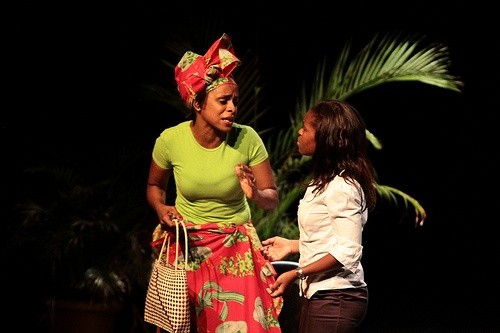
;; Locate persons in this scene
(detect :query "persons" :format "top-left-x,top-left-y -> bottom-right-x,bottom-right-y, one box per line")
147,33 -> 284,333
259,99 -> 377,333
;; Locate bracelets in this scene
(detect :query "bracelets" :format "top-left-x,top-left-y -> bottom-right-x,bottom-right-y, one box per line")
296,266 -> 303,278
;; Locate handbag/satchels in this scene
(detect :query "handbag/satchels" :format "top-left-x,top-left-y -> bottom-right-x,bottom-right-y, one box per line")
143,219 -> 191,333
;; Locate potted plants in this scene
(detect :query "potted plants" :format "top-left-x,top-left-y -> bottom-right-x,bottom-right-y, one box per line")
12,169 -> 152,333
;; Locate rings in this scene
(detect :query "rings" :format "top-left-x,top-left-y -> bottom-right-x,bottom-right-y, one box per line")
252,176 -> 257,182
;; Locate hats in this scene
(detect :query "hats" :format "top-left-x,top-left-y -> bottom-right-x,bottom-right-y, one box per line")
174,33 -> 241,106
